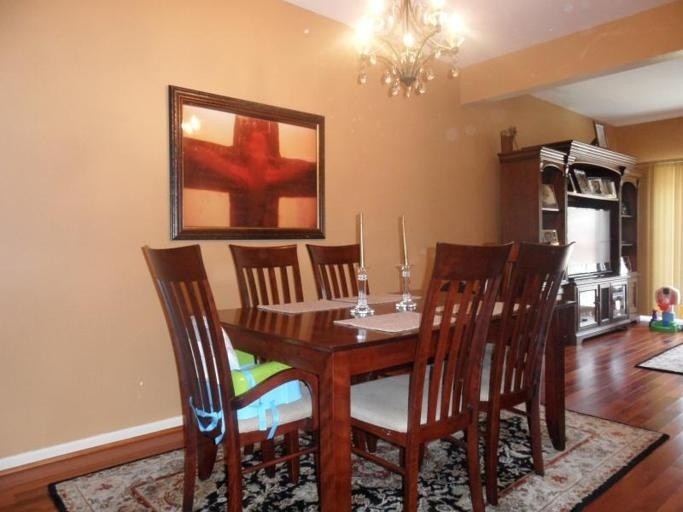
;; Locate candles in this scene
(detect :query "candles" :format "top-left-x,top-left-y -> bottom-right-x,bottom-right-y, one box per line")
400,215 -> 410,265
358,210 -> 368,272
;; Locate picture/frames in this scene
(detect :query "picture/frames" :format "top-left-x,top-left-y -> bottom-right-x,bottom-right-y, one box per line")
169,86 -> 327,239
592,120 -> 609,149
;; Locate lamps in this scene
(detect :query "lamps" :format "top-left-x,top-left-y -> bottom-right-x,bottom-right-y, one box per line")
354,0 -> 466,99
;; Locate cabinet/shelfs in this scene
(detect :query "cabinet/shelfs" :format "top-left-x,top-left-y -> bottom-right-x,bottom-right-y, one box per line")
497,141 -> 641,347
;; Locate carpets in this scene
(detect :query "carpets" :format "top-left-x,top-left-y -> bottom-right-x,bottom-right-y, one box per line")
47,403 -> 670,512
634,342 -> 683,375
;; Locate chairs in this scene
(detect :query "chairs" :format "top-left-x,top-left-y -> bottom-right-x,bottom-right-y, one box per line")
429,241 -> 577,507
228,245 -> 306,365
309,243 -> 370,300
350,241 -> 515,512
142,244 -> 319,512
